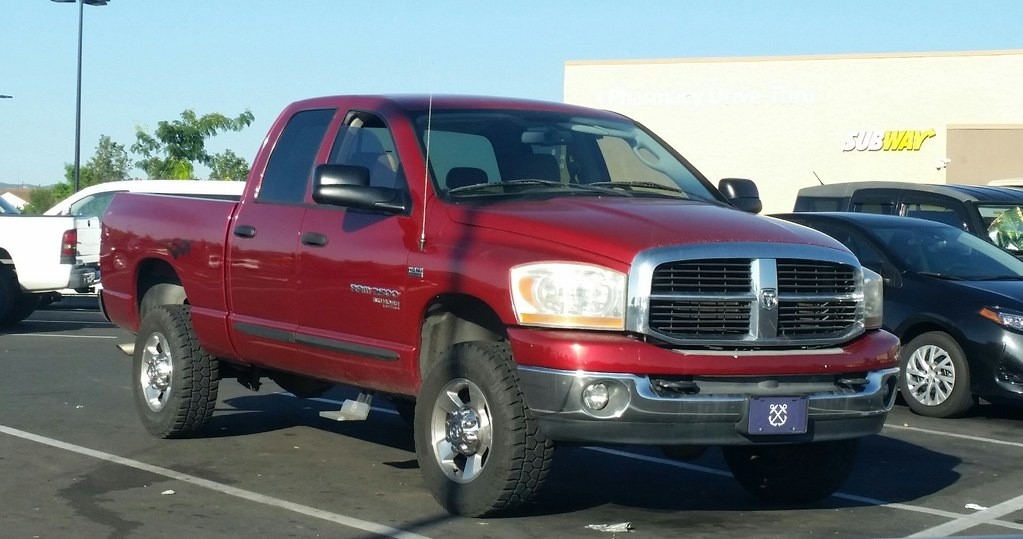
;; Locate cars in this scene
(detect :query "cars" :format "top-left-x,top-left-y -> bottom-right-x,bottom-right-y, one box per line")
762,211 -> 1023,416
0,194 -> 100,327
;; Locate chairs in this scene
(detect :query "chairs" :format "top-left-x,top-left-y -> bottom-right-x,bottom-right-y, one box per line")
446,167 -> 489,193
889,233 -> 931,271
515,155 -> 569,194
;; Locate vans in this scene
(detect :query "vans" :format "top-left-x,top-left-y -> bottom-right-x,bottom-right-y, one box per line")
39,179 -> 245,305
792,171 -> 1023,266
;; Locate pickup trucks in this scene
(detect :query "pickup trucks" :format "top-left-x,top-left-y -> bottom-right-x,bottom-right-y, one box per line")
97,91 -> 902,518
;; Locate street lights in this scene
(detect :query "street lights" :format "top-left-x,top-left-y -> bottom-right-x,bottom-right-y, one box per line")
53,0 -> 109,192
